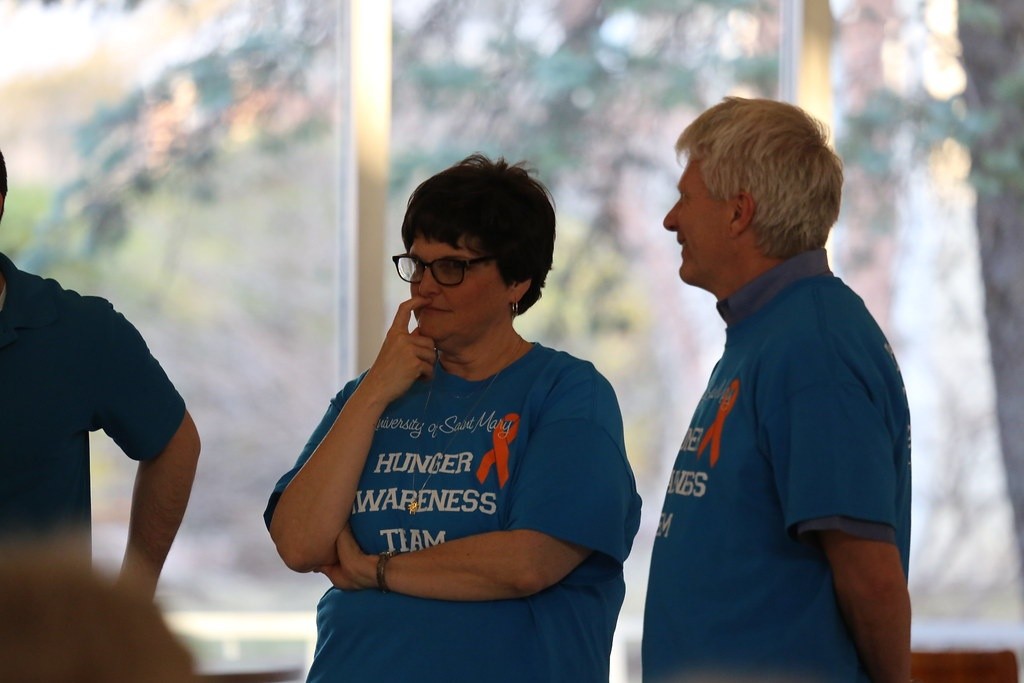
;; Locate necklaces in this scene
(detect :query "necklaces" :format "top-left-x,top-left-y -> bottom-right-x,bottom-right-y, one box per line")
409,333 -> 525,514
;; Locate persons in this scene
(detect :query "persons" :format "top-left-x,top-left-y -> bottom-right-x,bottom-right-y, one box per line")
0,153 -> 204,683
641,96 -> 913,683
263,155 -> 643,683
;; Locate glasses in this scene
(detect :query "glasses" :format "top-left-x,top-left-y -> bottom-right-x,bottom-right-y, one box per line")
392,253 -> 498,287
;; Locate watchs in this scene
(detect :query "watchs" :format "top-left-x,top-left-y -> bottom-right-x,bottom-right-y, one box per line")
375,548 -> 401,594
